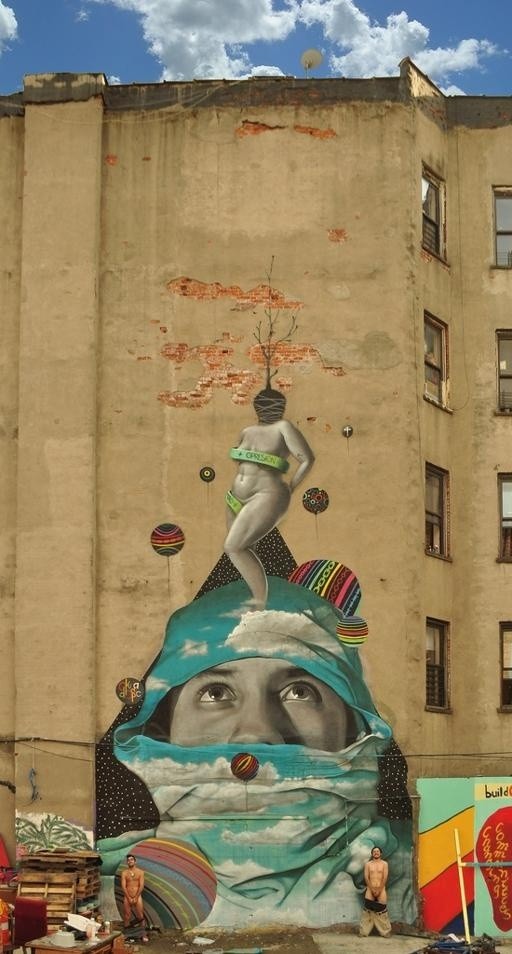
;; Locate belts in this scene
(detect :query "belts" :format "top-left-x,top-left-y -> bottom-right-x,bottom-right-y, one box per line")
124,918 -> 144,929
363,907 -> 388,915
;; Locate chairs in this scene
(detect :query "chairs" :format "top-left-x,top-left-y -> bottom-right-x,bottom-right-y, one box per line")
13,895 -> 47,946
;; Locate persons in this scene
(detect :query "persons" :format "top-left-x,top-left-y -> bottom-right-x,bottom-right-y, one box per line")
224,388 -> 314,613
121,855 -> 148,941
360,847 -> 391,938
168,658 -> 350,755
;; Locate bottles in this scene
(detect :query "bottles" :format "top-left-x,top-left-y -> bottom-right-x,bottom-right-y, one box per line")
88,918 -> 96,940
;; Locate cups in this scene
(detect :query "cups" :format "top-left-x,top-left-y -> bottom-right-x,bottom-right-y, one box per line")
104,921 -> 110,935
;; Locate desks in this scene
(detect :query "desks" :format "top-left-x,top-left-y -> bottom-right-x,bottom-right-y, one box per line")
22,929 -> 121,953
